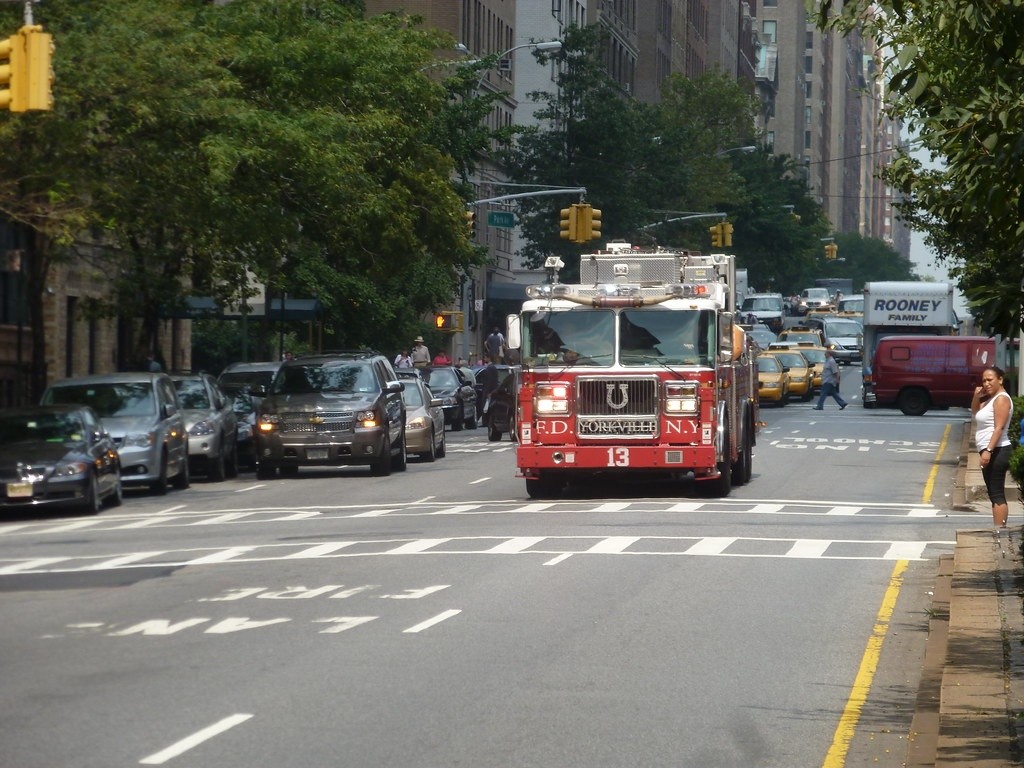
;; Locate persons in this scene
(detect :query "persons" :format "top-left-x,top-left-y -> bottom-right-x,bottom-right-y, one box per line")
394,350 -> 414,368
970,366 -> 1014,526
432,350 -> 451,366
410,336 -> 431,367
480,352 -> 499,427
486,326 -> 505,363
813,350 -> 848,410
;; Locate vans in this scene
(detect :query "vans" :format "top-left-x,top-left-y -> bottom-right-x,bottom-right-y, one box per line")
42,370 -> 188,496
739,292 -> 785,332
874,335 -> 1021,416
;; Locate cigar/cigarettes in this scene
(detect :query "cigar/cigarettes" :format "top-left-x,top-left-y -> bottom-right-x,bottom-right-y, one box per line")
981,466 -> 983,468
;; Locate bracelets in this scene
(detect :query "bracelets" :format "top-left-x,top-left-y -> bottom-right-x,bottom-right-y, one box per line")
985,448 -> 993,453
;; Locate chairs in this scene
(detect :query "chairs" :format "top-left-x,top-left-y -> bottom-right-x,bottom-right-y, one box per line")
94,387 -> 118,412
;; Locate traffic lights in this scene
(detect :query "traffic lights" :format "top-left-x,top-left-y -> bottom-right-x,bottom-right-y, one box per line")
710,224 -> 722,247
560,206 -> 577,240
825,245 -> 831,259
465,210 -> 476,238
436,314 -> 455,331
0,34 -> 28,113
586,204 -> 601,240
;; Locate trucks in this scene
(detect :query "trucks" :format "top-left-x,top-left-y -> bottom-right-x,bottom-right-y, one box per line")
816,278 -> 852,299
734,268 -> 748,311
862,283 -> 953,409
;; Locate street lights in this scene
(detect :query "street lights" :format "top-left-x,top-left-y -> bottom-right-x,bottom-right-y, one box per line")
463,42 -> 562,182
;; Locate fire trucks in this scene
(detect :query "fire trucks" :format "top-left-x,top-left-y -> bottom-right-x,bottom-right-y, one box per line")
504,239 -> 767,498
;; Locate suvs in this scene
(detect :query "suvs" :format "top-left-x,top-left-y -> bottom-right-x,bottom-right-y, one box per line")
168,350 -> 407,483
777,287 -> 863,365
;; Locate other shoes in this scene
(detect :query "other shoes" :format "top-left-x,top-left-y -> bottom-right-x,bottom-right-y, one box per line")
839,403 -> 848,410
813,407 -> 823,410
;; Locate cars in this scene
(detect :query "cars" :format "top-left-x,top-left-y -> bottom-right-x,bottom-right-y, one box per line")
740,324 -> 828,408
0,404 -> 121,515
393,364 -> 522,463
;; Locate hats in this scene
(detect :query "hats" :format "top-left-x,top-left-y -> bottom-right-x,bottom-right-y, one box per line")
414,336 -> 424,343
